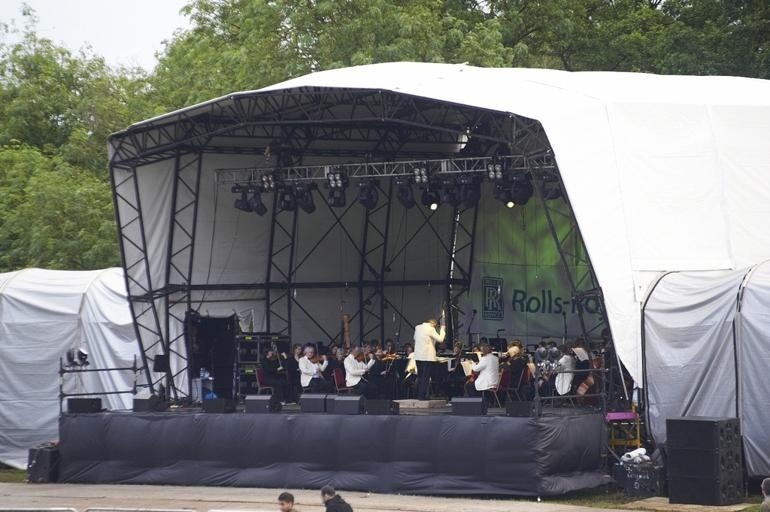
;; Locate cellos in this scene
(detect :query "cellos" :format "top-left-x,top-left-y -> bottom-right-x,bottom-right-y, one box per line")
576,340 -> 612,406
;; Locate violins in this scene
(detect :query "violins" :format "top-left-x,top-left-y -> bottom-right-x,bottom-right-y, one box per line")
381,352 -> 400,360
271,353 -> 285,361
355,353 -> 380,362
308,355 -> 331,362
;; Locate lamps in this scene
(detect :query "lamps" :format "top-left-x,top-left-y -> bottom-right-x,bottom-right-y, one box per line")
261,174 -> 275,189
422,189 -> 439,211
249,191 -> 268,215
356,184 -> 378,209
326,189 -> 345,208
295,186 -> 316,214
234,193 -> 251,213
395,185 -> 416,210
278,190 -> 296,211
327,173 -> 342,187
494,185 -> 515,209
412,167 -> 428,184
488,162 -> 502,179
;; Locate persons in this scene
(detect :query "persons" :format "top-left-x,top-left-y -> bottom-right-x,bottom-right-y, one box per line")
413,314 -> 448,400
278,491 -> 299,512
259,338 -> 417,406
601,327 -> 610,343
320,484 -> 354,511
760,477 -> 770,511
455,339 -> 612,407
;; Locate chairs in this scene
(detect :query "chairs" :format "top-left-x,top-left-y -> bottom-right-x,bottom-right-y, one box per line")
490,363 -> 534,407
255,368 -> 275,396
333,368 -> 354,394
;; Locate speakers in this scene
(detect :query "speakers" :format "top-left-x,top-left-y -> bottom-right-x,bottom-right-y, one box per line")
299,392 -> 400,415
204,398 -> 236,413
132,399 -> 150,411
28,445 -> 59,484
451,397 -> 489,416
67,397 -> 101,413
505,400 -> 534,417
244,395 -> 282,413
665,416 -> 744,506
188,316 -> 236,397
